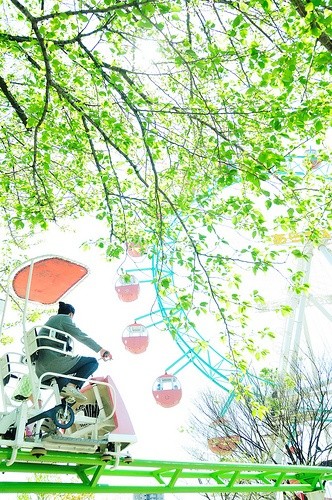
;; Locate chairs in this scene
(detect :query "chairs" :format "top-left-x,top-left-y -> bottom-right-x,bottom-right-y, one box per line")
0,351 -> 30,408
21,326 -> 74,389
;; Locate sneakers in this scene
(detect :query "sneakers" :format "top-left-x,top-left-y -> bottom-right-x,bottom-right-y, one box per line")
60,386 -> 87,402
40,418 -> 63,436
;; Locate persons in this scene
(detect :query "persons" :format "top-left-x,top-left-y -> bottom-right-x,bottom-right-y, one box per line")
34,301 -> 113,439
